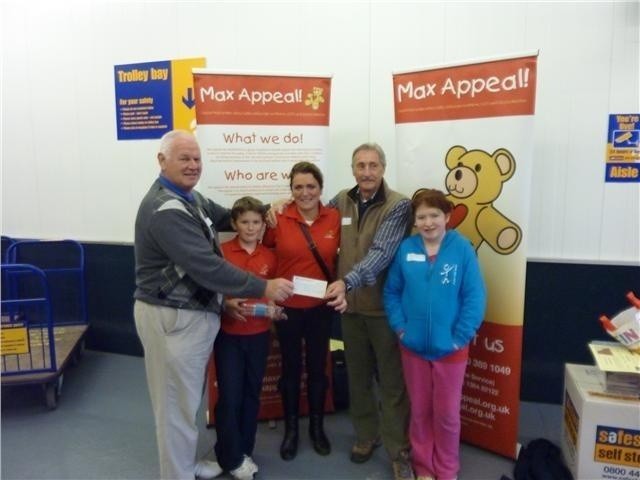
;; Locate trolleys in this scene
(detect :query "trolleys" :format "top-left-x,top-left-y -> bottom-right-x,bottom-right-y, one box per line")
0,237 -> 92,413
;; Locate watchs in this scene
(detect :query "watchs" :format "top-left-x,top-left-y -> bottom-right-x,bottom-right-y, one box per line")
343,278 -> 352,292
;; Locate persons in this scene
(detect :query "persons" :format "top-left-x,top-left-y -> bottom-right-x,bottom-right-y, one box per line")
132,128 -> 292,480
266,142 -> 413,480
215,196 -> 289,480
263,161 -> 341,460
383,189 -> 486,480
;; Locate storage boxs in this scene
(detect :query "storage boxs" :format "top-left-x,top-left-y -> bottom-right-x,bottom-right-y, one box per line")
559,361 -> 640,480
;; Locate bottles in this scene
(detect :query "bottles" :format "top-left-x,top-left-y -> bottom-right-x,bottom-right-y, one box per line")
241,303 -> 282,317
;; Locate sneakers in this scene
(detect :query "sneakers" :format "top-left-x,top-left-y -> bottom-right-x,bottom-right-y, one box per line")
392,448 -> 416,480
194,458 -> 224,479
350,434 -> 384,464
230,453 -> 258,479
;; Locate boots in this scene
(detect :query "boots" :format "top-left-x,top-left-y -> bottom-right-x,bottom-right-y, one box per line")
309,411 -> 332,456
279,412 -> 300,461
